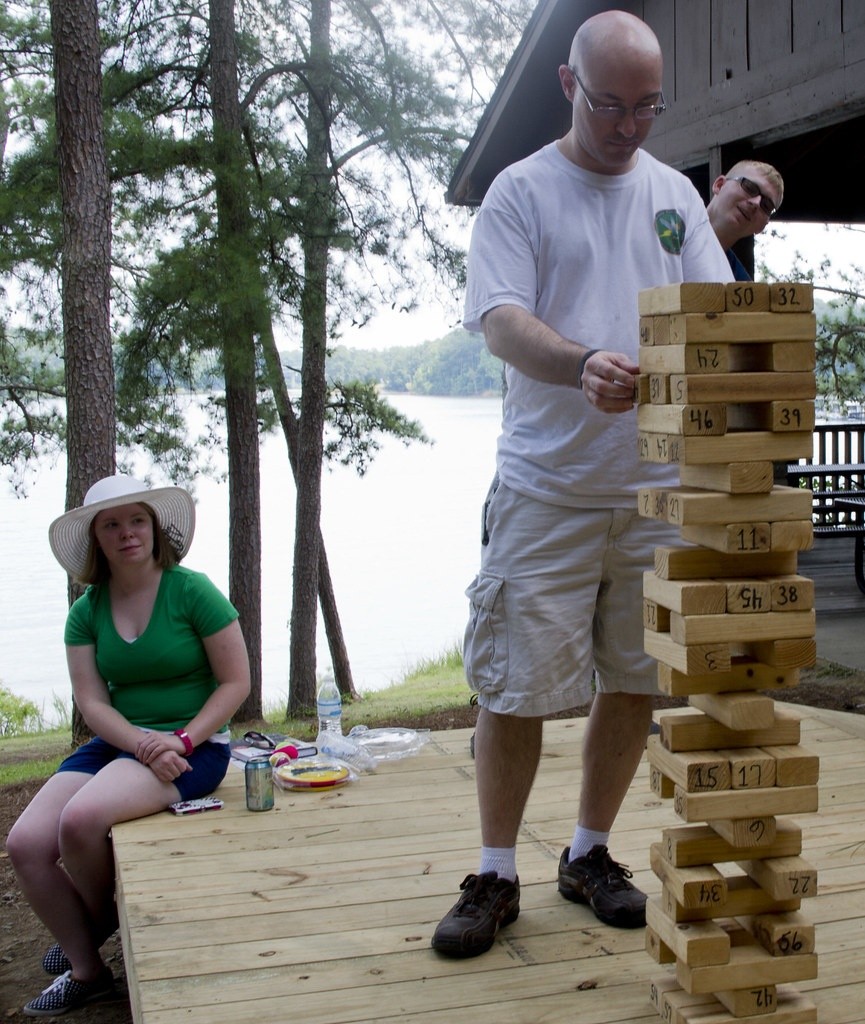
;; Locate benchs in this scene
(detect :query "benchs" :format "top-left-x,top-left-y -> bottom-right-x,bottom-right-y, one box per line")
812,488 -> 865,595
787,463 -> 865,477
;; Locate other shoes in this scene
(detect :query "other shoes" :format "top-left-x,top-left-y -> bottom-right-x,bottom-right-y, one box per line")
645,720 -> 661,734
43,941 -> 73,974
24,965 -> 115,1016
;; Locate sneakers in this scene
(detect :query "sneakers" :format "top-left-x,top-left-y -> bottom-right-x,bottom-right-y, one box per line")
558,845 -> 648,919
431,870 -> 520,959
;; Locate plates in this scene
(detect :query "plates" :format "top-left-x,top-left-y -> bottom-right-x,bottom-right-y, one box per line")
360,727 -> 418,749
277,761 -> 349,792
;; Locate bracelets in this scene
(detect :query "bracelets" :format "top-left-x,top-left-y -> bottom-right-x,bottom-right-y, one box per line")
173,729 -> 193,756
578,349 -> 603,389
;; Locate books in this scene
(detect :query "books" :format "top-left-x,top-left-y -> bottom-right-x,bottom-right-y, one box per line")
231,734 -> 318,763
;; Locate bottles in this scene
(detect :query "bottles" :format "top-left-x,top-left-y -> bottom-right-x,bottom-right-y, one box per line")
348,726 -> 368,749
317,675 -> 342,733
315,730 -> 378,773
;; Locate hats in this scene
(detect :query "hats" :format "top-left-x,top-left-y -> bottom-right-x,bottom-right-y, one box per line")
48,475 -> 196,585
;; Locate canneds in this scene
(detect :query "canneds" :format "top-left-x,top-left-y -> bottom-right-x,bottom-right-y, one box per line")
244,756 -> 275,811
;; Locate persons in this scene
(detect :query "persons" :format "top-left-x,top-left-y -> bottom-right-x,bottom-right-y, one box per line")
5,474 -> 251,1015
708,160 -> 783,282
432,8 -> 736,960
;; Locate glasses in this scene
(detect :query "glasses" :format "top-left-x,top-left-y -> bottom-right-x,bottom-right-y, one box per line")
727,176 -> 777,218
573,72 -> 666,120
244,731 -> 276,749
